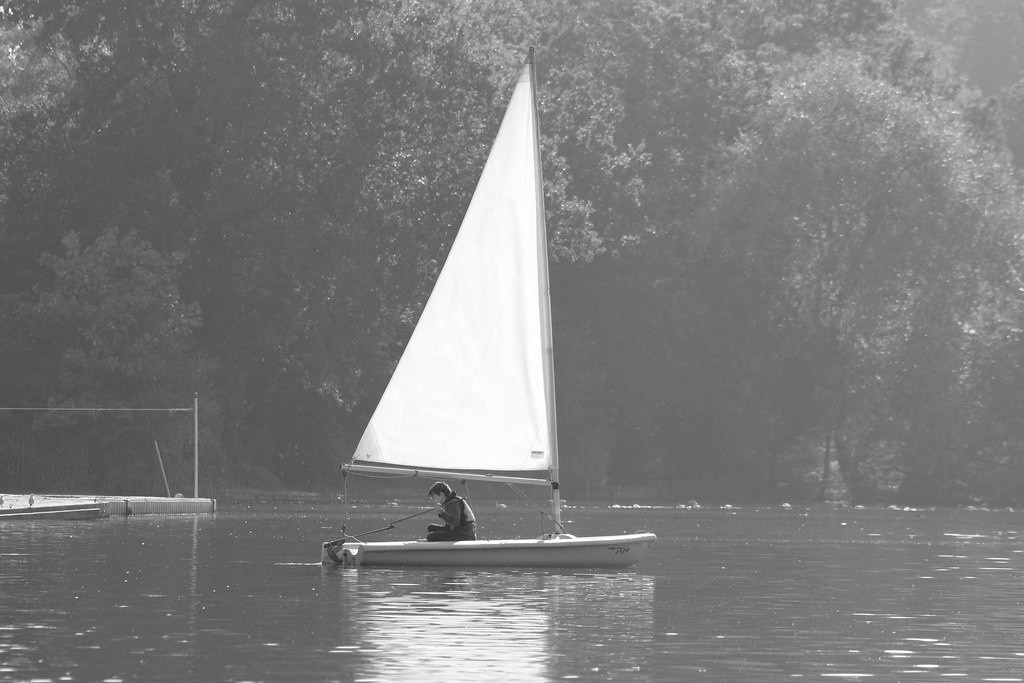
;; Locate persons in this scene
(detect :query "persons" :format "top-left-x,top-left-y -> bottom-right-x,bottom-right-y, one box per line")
426,482 -> 477,542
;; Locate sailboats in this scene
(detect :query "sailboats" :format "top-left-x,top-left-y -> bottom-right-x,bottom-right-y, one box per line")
304,53 -> 671,577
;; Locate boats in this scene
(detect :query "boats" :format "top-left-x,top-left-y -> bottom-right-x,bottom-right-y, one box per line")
0,491 -> 220,520
